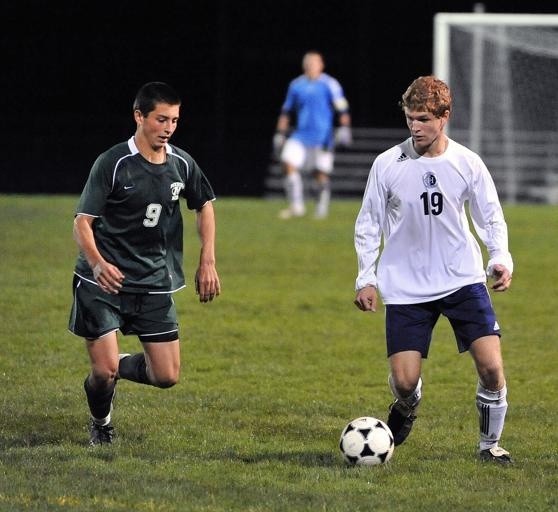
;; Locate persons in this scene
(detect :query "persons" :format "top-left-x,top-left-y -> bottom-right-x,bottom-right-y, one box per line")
72,81 -> 222,447
352,75 -> 517,461
273,51 -> 353,220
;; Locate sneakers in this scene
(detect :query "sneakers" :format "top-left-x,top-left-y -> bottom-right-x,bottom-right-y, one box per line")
479,447 -> 513,467
280,205 -> 305,218
91,419 -> 115,446
387,397 -> 417,446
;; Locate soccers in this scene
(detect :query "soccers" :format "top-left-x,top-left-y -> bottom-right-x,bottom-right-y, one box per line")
338,416 -> 395,467
